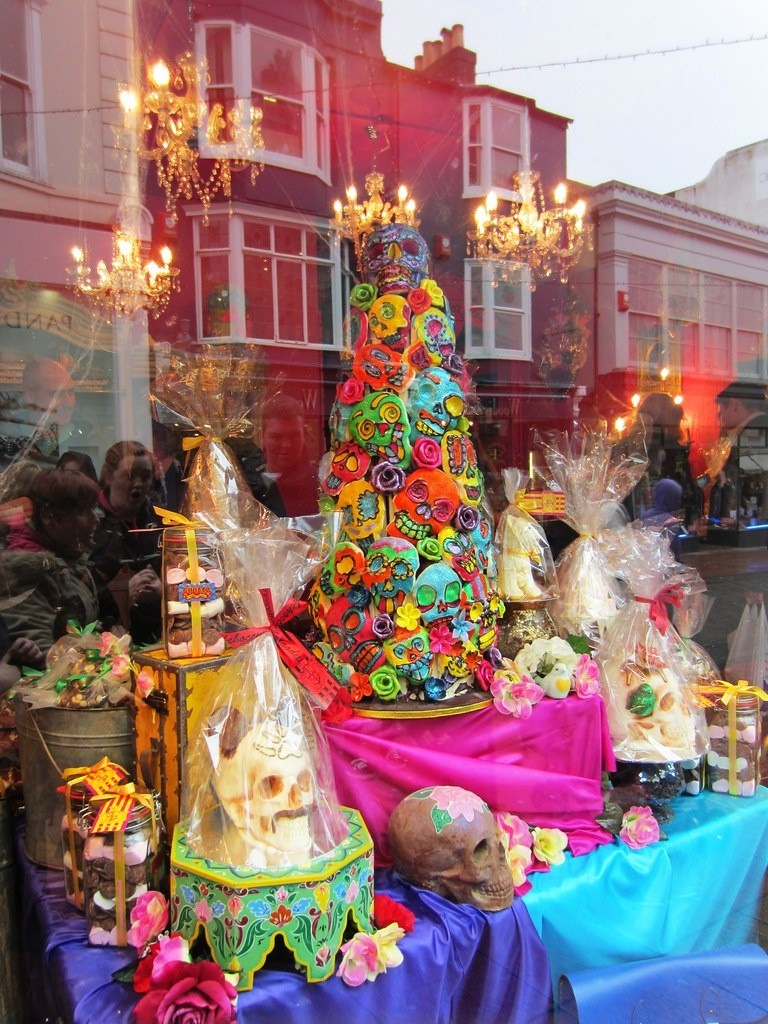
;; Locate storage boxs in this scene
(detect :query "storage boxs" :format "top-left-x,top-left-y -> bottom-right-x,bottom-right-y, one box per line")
128,646 -> 301,857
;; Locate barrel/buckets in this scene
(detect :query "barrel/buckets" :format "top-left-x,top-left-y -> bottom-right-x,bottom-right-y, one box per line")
14,697 -> 136,871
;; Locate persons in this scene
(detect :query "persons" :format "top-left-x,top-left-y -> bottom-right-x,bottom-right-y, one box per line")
1,379 -> 768,676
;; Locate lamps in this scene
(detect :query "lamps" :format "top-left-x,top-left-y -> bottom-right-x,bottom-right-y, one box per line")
64,217 -> 182,329
465,167 -> 595,294
110,0 -> 266,226
327,124 -> 424,276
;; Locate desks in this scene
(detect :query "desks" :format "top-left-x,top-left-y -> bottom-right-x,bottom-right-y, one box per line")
11,783 -> 768,1024
